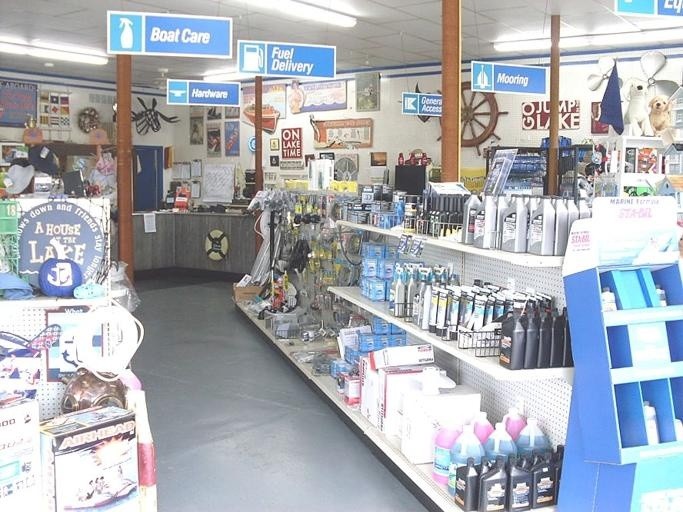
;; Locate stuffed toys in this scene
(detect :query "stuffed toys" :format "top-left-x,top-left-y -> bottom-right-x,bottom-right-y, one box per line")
647,95 -> 674,137
618,77 -> 655,137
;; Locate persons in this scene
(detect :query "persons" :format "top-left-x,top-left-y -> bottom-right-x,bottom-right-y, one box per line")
288,80 -> 305,113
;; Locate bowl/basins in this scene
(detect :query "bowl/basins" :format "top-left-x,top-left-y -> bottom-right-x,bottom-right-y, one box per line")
61,374 -> 129,413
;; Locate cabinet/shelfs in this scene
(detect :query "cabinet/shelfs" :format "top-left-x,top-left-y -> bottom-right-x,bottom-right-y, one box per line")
560,265 -> 683,467
306,218 -> 575,510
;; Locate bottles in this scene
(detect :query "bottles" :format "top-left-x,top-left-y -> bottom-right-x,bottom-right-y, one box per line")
497,306 -> 576,369
656,284 -> 667,307
421,188 -> 467,236
409,154 -> 417,165
393,271 -> 458,329
464,189 -> 591,255
601,286 -> 618,315
644,399 -> 660,445
398,153 -> 404,165
421,152 -> 427,165
455,445 -> 566,512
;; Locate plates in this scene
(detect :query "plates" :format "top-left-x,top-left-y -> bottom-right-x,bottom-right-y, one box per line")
79,109 -> 100,133
247,136 -> 255,152
89,128 -> 107,144
23,128 -> 42,144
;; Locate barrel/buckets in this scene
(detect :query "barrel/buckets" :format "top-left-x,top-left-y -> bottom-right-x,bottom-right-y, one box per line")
431,407 -> 564,511
496,306 -> 572,371
460,189 -> 594,256
